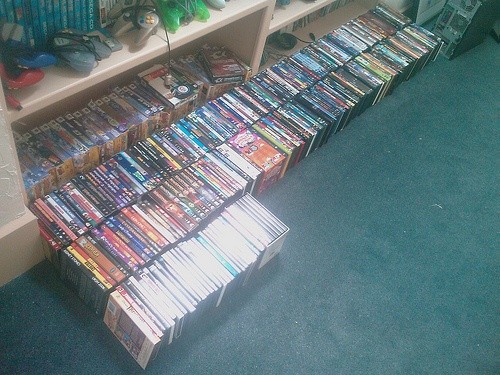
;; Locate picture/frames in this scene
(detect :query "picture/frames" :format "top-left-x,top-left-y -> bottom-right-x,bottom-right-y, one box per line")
414,0 -> 449,27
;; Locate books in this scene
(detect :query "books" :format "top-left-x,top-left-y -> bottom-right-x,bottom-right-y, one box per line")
0,1 -> 444,370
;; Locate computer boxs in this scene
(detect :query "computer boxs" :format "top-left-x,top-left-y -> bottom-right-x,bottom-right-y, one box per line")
432,1 -> 500,61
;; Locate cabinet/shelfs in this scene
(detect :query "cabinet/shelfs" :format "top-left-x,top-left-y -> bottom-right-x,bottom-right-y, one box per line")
1,1 -> 415,204
